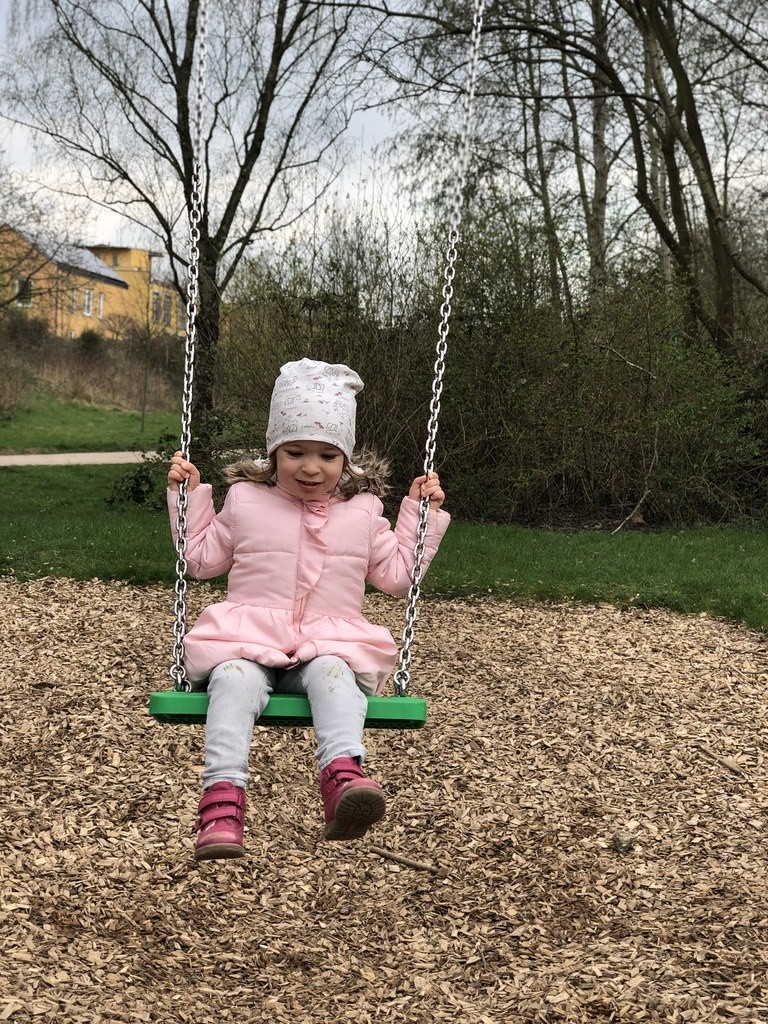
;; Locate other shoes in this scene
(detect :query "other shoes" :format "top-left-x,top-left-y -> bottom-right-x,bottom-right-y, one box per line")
192,781 -> 246,859
317,757 -> 387,842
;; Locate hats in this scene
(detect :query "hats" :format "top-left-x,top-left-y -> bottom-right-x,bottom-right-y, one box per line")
264,358 -> 364,462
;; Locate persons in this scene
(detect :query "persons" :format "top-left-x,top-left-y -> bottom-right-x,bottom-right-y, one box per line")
166,356 -> 450,860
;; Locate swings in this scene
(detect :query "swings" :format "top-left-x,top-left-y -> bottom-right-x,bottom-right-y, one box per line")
148,0 -> 487,728
